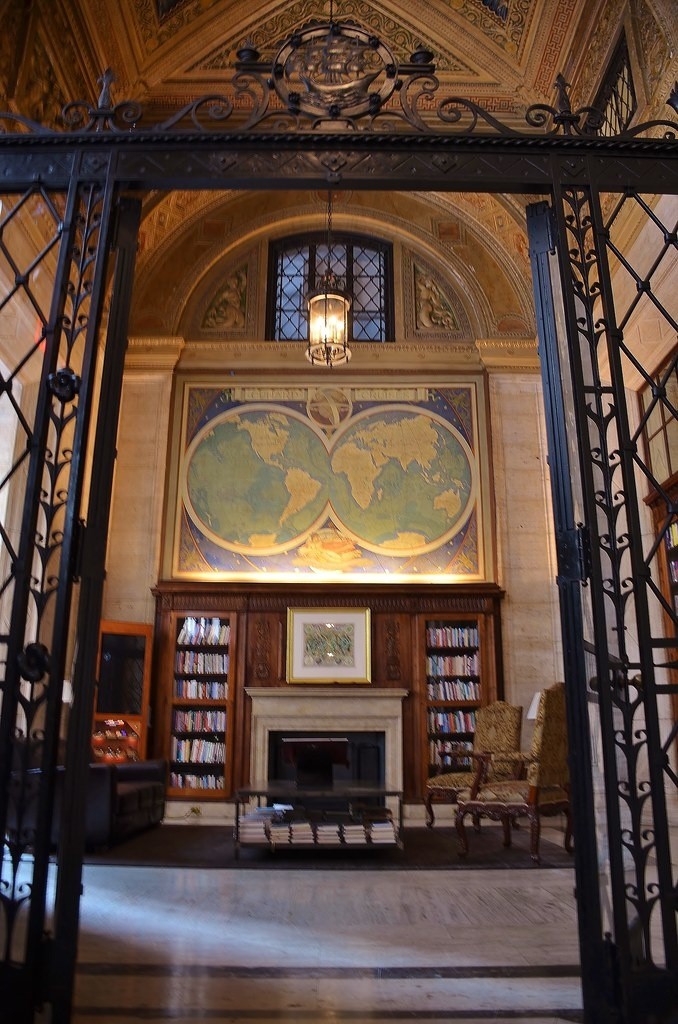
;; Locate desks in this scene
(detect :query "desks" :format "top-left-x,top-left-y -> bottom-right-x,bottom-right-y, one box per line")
234,788 -> 405,845
157,607 -> 238,797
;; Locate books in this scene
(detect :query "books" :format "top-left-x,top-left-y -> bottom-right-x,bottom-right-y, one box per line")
429,740 -> 475,766
427,710 -> 477,734
425,654 -> 480,677
177,616 -> 231,646
171,736 -> 226,764
174,679 -> 227,700
176,650 -> 229,675
428,679 -> 481,701
426,625 -> 479,648
237,804 -> 398,844
170,771 -> 224,790
175,711 -> 226,732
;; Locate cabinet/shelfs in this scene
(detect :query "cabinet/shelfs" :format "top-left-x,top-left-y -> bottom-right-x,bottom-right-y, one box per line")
411,613 -> 487,800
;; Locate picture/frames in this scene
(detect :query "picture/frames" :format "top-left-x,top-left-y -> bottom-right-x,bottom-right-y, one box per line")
286,605 -> 372,685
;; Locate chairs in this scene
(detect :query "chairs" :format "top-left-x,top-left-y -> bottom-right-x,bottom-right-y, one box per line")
423,698 -> 527,834
454,680 -> 574,862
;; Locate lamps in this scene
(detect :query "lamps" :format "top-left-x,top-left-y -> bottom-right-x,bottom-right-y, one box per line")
303,187 -> 354,369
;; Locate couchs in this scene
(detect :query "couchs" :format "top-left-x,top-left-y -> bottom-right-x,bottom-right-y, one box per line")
1,735 -> 169,852
